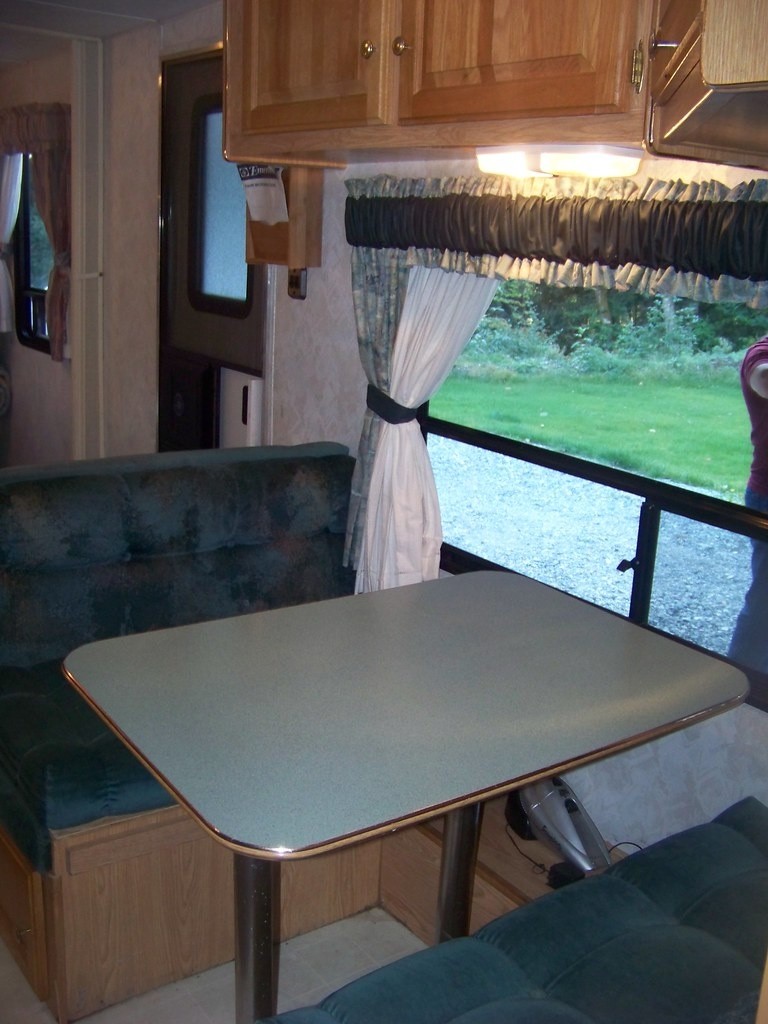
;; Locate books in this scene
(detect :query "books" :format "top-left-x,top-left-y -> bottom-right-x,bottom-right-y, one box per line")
237,163 -> 289,225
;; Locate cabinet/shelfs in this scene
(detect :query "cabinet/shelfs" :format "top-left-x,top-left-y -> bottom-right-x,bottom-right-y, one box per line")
219,1 -> 767,171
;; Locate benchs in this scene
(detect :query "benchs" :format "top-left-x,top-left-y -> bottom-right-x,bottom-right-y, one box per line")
245,798 -> 767,1024
0,439 -> 356,1024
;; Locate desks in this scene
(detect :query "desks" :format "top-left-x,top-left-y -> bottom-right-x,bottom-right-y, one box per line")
60,570 -> 751,1024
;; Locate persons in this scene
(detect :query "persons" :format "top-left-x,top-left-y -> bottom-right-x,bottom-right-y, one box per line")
727,334 -> 767,675
